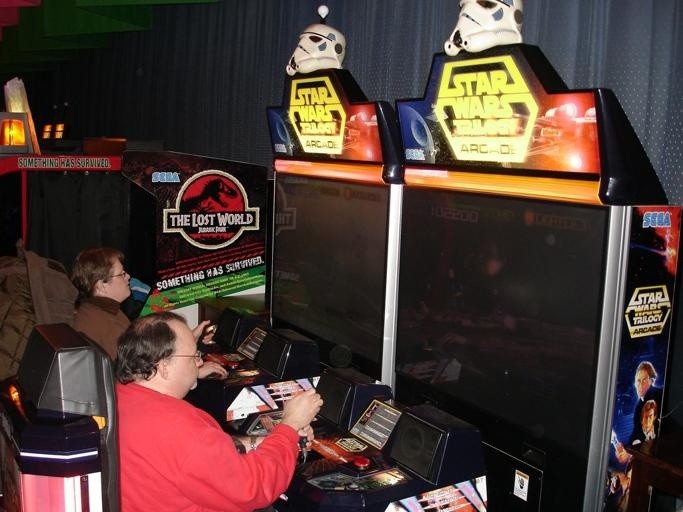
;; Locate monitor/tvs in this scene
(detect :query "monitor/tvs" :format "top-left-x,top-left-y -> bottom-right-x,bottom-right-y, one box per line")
271,172 -> 392,383
392,171 -> 610,471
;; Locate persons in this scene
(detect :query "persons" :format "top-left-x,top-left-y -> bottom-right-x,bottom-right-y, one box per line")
616,399 -> 662,478
70,244 -> 133,361
626,362 -> 663,451
114,312 -> 323,512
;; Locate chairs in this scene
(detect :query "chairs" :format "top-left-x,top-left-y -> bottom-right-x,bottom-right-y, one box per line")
0,319 -> 121,511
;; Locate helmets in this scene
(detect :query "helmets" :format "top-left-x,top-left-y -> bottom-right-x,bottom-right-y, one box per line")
286,24 -> 347,76
444,0 -> 522,57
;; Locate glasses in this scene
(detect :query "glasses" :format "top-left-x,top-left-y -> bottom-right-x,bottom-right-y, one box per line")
167,350 -> 201,360
109,269 -> 126,278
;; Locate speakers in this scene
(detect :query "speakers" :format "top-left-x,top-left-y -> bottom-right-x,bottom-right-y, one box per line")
255,332 -> 315,379
213,306 -> 260,349
387,405 -> 475,490
310,361 -> 393,433
